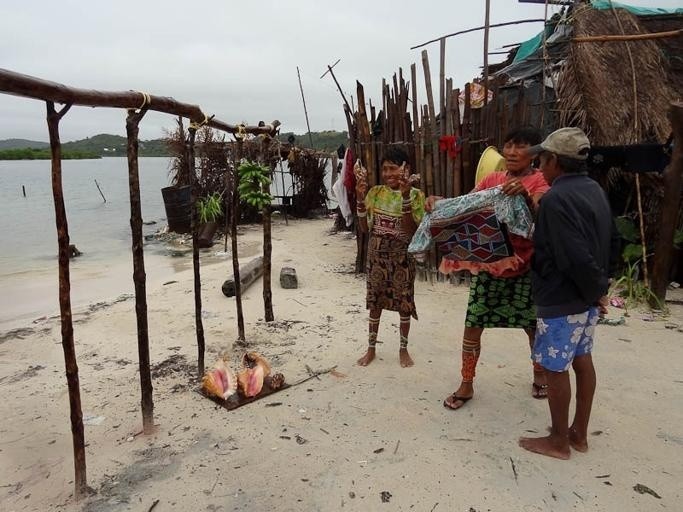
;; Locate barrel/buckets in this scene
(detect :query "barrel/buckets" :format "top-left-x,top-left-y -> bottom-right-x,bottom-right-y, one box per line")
162,185 -> 192,233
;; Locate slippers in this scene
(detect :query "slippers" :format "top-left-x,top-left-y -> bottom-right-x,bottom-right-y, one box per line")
532,382 -> 549,399
442,392 -> 473,410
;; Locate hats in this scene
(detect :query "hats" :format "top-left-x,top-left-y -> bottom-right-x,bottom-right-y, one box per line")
527,125 -> 591,161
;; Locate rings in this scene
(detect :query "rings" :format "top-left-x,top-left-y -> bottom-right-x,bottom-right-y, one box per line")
510,182 -> 516,187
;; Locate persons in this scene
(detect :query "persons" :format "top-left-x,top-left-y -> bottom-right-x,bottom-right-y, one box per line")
515,127 -> 614,462
423,122 -> 552,408
351,144 -> 427,369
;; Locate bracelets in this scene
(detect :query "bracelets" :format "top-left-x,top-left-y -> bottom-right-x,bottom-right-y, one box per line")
400,197 -> 414,216
356,199 -> 366,218
